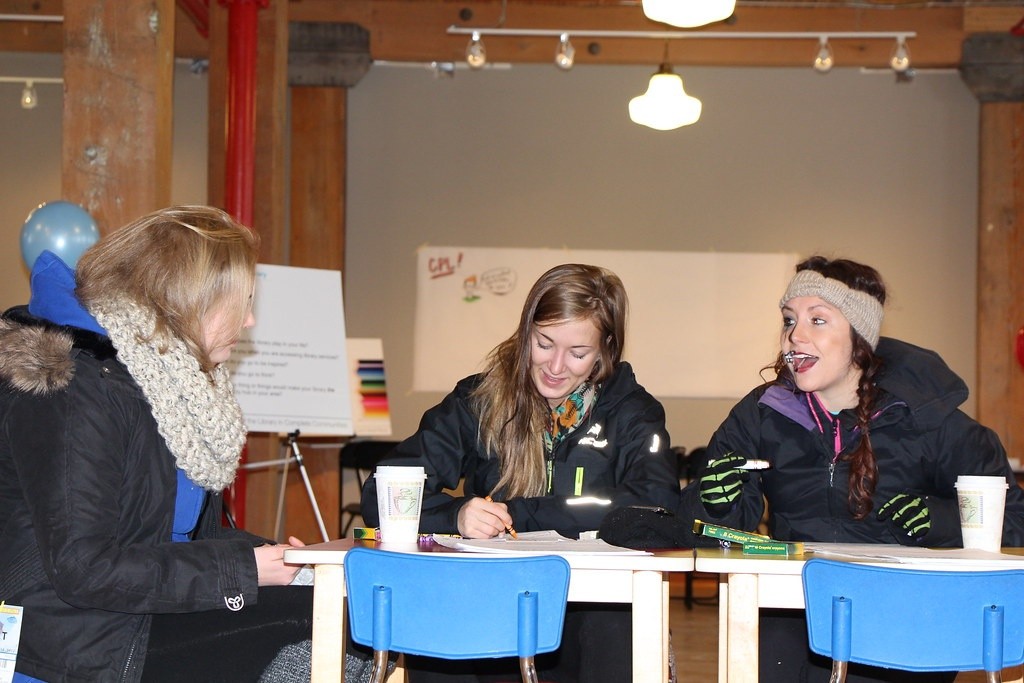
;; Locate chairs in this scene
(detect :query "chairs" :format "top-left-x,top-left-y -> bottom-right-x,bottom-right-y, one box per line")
801,558 -> 1024,683
343,546 -> 571,682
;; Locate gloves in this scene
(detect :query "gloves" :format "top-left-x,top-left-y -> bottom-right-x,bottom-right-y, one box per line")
698,450 -> 750,519
876,493 -> 931,539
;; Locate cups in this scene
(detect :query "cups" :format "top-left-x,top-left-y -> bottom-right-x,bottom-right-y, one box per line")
954,476 -> 1009,552
372,465 -> 427,542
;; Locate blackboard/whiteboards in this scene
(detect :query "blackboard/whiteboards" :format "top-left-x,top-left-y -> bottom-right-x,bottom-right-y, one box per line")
340,60 -> 980,532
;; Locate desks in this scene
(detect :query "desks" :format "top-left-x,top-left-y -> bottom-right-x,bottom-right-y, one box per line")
284,533 -> 1024,683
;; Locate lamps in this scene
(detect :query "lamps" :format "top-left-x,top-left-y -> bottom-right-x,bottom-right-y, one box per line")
443,0 -> 917,72
627,42 -> 737,131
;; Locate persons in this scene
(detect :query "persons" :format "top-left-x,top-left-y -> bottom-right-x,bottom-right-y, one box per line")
358,262 -> 681,683
0,205 -> 400,683
698,257 -> 1024,683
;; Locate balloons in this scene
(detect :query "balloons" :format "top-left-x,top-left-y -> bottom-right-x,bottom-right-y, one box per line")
20,200 -> 100,272
